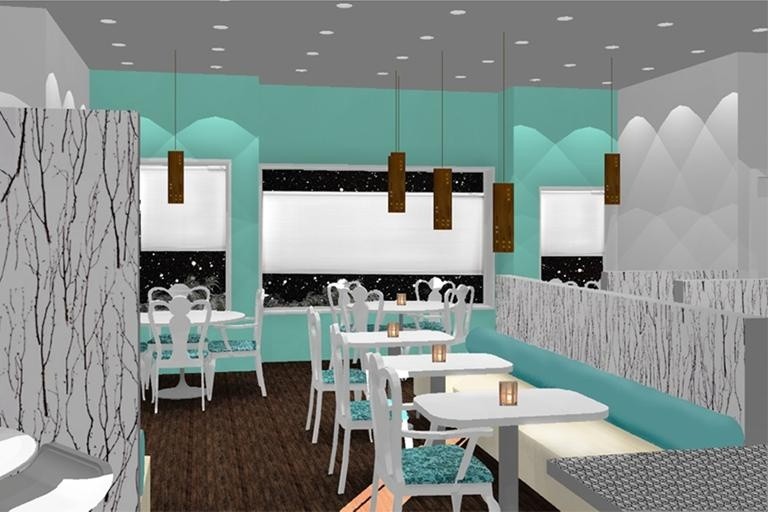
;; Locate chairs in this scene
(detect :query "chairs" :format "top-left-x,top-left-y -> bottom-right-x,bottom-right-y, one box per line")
303,277 -> 503,511
140,282 -> 268,414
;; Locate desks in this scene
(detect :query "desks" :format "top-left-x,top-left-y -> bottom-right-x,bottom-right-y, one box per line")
381,354 -> 610,511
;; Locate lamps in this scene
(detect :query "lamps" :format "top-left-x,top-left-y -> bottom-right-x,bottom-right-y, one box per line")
499,380 -> 519,405
603,56 -> 620,206
386,32 -> 514,252
168,50 -> 184,204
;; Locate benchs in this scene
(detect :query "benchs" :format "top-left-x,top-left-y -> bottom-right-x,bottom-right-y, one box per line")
413,327 -> 745,512
